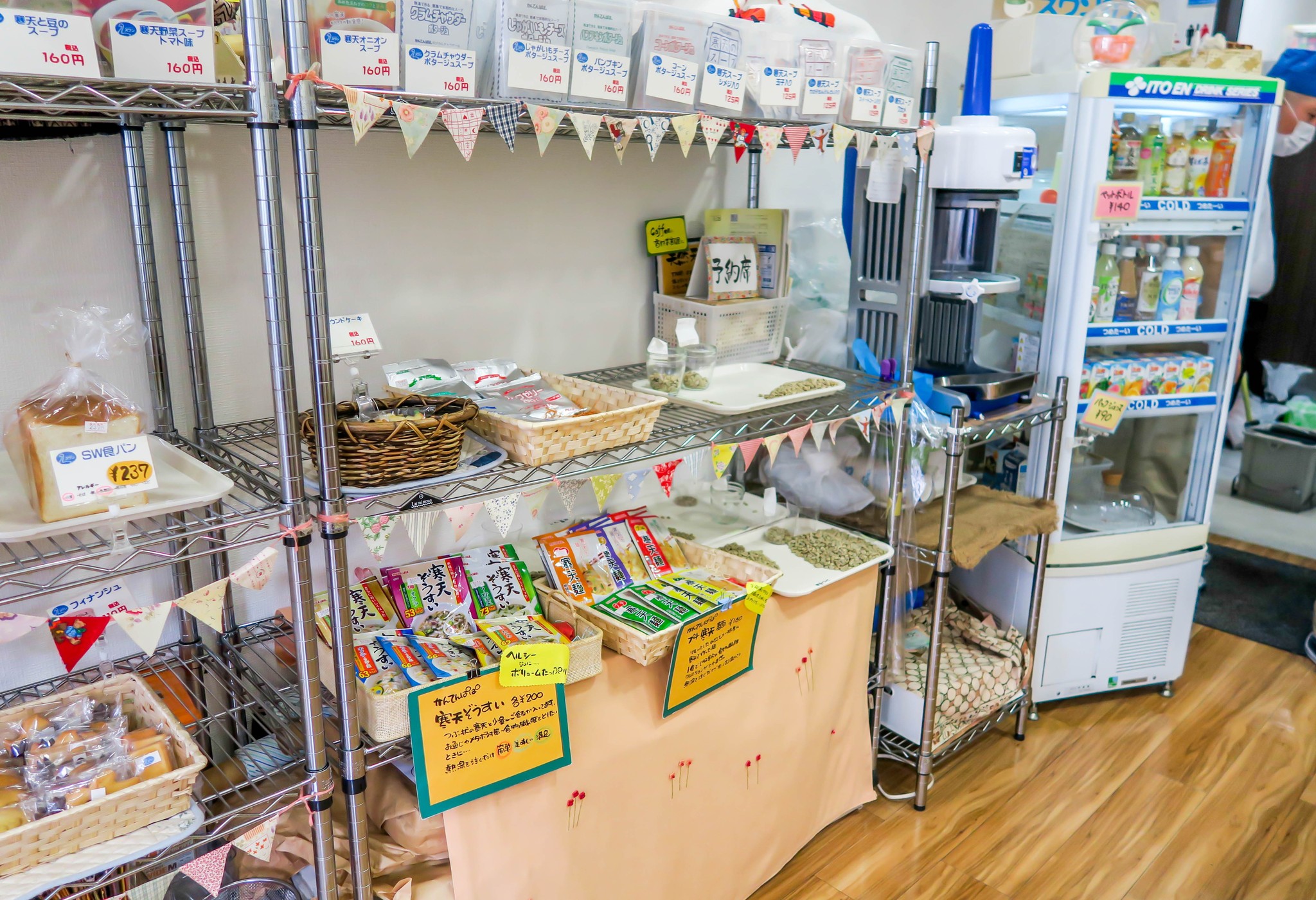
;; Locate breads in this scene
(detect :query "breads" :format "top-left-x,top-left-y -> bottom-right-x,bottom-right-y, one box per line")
16,393 -> 151,524
0,689 -> 171,836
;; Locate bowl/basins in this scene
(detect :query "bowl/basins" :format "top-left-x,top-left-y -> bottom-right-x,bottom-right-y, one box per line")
1235,422 -> 1316,511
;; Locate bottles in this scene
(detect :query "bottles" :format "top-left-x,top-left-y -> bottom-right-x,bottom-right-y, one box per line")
1023,112 -> 1238,325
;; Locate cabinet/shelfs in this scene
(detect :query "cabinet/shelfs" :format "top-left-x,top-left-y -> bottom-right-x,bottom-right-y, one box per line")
157,0 -> 937,900
843,365 -> 1068,812
0,0 -> 339,900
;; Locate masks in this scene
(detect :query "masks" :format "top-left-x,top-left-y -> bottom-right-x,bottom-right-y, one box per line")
1273,97 -> 1316,158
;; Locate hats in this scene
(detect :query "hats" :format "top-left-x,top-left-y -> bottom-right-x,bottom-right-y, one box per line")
1265,48 -> 1316,97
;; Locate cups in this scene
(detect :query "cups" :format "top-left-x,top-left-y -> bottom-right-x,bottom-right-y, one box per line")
681,344 -> 718,391
646,347 -> 687,397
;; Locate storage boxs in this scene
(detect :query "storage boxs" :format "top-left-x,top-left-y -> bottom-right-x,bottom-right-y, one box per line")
1230,422 -> 1316,513
0,674 -> 207,879
298,291 -> 896,744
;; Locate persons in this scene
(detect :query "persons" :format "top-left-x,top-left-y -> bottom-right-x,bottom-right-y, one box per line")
1085,48 -> 1315,588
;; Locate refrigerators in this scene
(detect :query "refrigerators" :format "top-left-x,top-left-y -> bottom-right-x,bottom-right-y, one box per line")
977,63 -> 1283,719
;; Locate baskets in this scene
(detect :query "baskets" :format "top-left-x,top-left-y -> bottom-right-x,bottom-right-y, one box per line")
460,368 -> 668,466
299,394 -> 480,489
0,671 -> 208,878
529,535 -> 782,666
